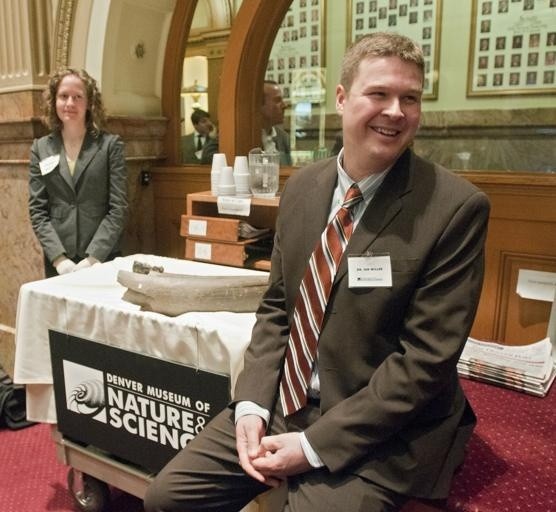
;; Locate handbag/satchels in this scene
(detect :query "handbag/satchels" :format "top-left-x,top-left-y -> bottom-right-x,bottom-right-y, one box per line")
118,261 -> 269,316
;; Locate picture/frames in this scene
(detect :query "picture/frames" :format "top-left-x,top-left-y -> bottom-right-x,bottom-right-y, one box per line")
464,0 -> 556,96
345,0 -> 442,100
259,0 -> 328,108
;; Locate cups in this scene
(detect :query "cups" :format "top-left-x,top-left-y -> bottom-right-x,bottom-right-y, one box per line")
209,151 -> 248,196
248,152 -> 280,198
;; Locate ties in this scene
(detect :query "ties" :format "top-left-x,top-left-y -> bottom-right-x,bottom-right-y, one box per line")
279,184 -> 363,419
197,135 -> 203,150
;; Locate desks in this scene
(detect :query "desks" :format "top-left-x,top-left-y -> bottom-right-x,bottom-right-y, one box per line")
13,249 -> 271,512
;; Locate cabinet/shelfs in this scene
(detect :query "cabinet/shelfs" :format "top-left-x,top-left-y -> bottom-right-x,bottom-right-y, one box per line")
185,190 -> 285,272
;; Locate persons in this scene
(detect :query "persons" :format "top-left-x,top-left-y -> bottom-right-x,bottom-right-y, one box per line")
142,32 -> 491,512
26,66 -> 130,275
182,107 -> 217,164
259,80 -> 292,166
